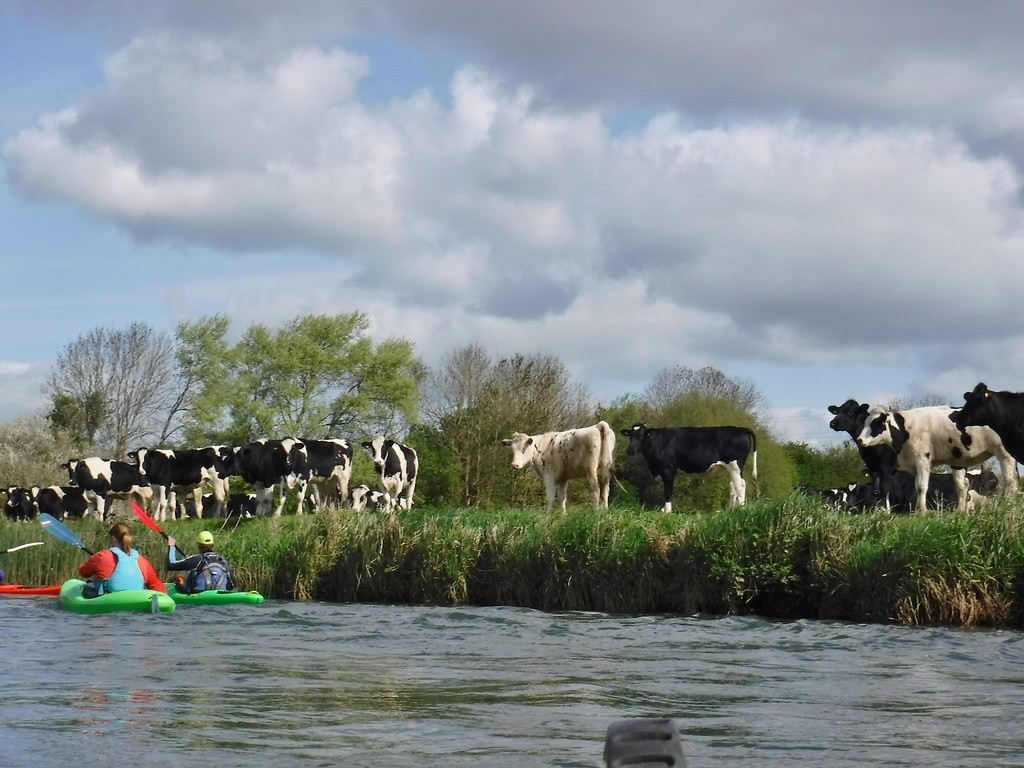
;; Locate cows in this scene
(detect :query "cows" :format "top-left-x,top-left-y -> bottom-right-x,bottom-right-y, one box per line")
620,422 -> 758,514
502,421 -> 615,513
0,436 -> 419,523
793,383 -> 1024,515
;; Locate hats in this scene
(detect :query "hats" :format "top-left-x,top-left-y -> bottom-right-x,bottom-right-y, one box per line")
197,531 -> 214,544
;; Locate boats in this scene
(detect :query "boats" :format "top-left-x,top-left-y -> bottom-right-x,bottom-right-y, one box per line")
0,584 -> 61,599
162,582 -> 264,605
60,578 -> 177,613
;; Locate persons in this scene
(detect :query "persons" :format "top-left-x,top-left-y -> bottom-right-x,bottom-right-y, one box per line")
78,522 -> 168,595
166,531 -> 237,594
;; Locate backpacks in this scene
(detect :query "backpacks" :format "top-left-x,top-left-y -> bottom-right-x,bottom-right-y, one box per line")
192,554 -> 227,593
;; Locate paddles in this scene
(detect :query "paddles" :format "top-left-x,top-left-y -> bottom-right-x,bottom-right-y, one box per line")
39,512 -> 97,556
0,541 -> 44,556
130,499 -> 187,557
